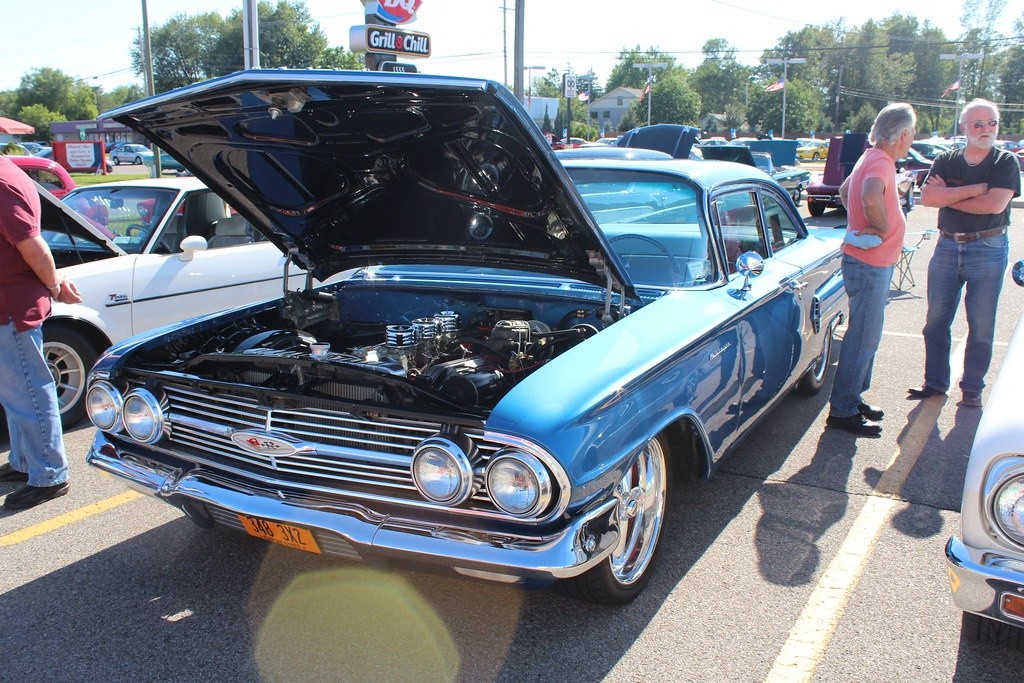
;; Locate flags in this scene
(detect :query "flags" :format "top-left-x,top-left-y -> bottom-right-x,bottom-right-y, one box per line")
943,77 -> 961,94
579,90 -> 589,101
639,82 -> 650,101
765,76 -> 784,92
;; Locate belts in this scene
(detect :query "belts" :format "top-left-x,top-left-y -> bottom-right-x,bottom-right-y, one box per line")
940,225 -> 1008,244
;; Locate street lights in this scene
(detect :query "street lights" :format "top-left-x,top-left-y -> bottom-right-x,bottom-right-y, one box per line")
580,75 -> 598,140
524,65 -> 546,120
938,52 -> 984,140
631,63 -> 669,126
766,58 -> 807,141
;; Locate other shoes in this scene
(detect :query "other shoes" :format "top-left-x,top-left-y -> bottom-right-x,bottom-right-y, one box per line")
4,481 -> 70,510
963,390 -> 981,406
825,412 -> 882,435
0,462 -> 29,483
857,398 -> 884,420
909,385 -> 946,398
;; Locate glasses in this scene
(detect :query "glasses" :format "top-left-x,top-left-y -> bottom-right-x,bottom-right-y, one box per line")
964,119 -> 998,128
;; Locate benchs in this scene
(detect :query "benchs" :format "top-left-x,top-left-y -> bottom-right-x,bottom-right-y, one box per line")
604,228 -> 764,286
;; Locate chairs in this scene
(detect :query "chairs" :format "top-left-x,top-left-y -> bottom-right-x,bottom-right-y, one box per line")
890,206 -> 938,292
138,215 -> 185,253
208,217 -> 255,248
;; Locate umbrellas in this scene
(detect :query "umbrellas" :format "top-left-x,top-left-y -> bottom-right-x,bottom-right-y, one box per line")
0,116 -> 36,136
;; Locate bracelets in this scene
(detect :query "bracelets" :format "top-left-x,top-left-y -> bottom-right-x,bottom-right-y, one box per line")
49,282 -> 58,289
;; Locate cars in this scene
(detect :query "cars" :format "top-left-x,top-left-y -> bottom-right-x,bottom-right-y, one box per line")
84,67 -> 838,607
941,258 -> 1024,634
540,121 -> 1024,218
100,140 -> 186,174
26,172 -> 320,432
133,197 -> 241,226
0,141 -> 55,162
6,153 -> 111,225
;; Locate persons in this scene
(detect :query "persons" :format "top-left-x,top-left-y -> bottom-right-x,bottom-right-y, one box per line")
824,104 -> 918,434
0,149 -> 82,509
905,97 -> 1021,408
